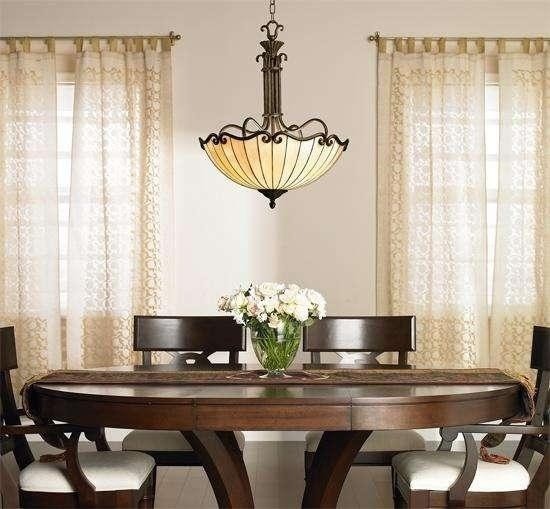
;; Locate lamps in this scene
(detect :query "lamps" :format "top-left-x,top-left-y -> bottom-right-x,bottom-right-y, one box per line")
197,0 -> 350,209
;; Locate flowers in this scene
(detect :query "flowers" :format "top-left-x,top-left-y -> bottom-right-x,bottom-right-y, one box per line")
217,282 -> 327,369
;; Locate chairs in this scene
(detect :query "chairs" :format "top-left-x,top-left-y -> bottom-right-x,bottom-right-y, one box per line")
3,316 -> 550,508
